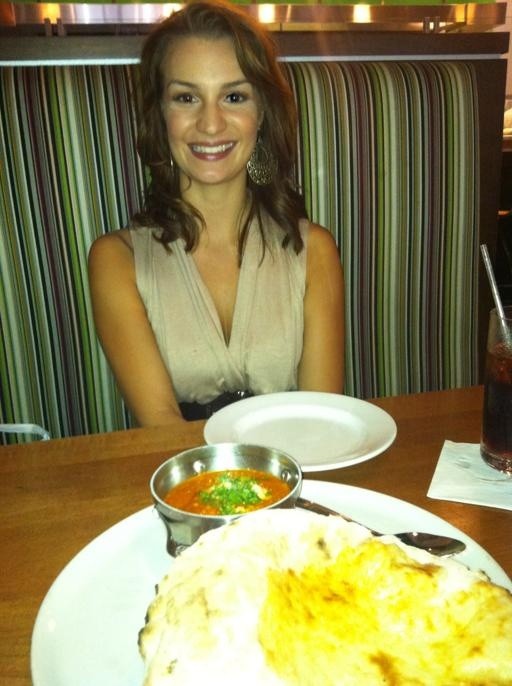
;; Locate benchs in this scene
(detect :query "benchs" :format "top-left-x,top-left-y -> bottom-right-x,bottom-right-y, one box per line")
0,32 -> 508,444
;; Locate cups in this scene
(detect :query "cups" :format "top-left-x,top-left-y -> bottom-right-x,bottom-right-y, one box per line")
478,305 -> 511,471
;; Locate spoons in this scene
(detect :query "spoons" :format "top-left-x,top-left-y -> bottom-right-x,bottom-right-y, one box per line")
294,496 -> 467,559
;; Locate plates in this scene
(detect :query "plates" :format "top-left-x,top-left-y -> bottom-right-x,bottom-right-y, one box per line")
203,391 -> 398,475
30,479 -> 511,686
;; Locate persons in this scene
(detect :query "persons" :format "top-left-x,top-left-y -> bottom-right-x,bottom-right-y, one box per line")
82,0 -> 346,429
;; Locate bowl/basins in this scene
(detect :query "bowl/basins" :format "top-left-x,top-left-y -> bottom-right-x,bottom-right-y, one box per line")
150,442 -> 305,560
